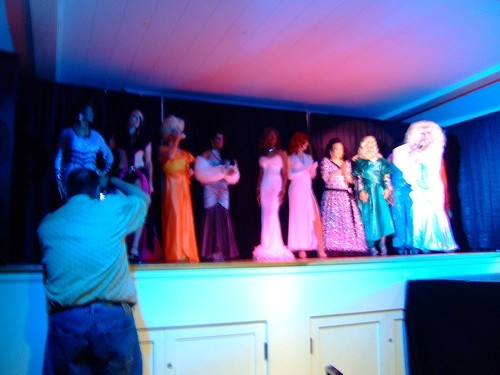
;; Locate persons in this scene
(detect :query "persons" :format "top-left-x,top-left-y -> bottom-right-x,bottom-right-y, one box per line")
253,128 -> 295,261
158,117 -> 200,263
354,121 -> 458,255
320,138 -> 368,251
287,134 -> 326,258
109,108 -> 153,263
55,103 -> 113,201
195,131 -> 239,260
38,168 -> 151,375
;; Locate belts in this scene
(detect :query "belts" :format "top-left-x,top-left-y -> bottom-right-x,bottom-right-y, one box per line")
48,300 -> 135,318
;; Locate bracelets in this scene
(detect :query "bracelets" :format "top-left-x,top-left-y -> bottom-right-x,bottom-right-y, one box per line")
55,173 -> 61,181
107,176 -> 114,189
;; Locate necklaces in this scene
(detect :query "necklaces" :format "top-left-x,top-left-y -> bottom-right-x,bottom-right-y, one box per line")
267,147 -> 275,152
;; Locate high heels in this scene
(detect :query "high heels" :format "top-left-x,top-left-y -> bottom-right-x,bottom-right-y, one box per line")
129,247 -> 139,263
398,247 -> 420,255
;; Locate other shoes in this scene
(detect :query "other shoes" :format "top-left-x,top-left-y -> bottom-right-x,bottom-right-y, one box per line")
298,250 -> 327,259
370,243 -> 387,255
